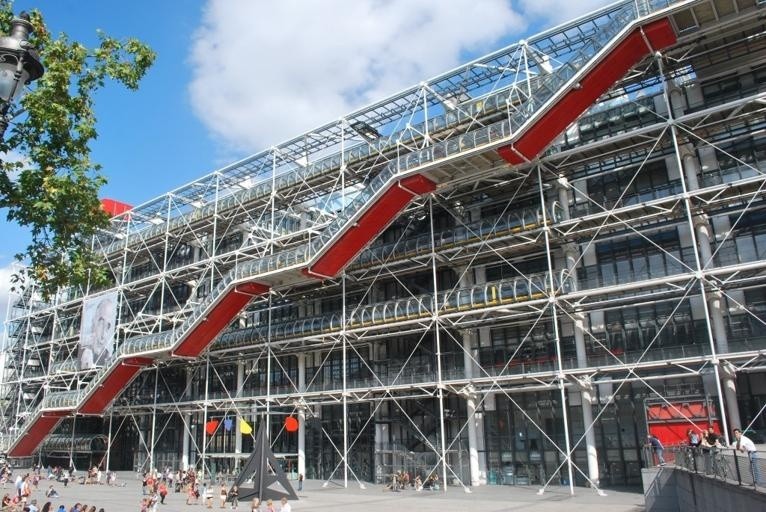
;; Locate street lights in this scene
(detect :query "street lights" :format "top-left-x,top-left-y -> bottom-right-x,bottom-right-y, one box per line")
0,11 -> 45,142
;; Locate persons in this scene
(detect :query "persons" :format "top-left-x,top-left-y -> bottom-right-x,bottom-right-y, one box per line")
701,429 -> 715,473
646,434 -> 666,466
391,469 -> 438,491
78,297 -> 117,368
141,463 -> 293,512
0,462 -> 116,512
708,425 -> 720,471
687,430 -> 702,457
297,474 -> 303,491
733,427 -> 760,486
